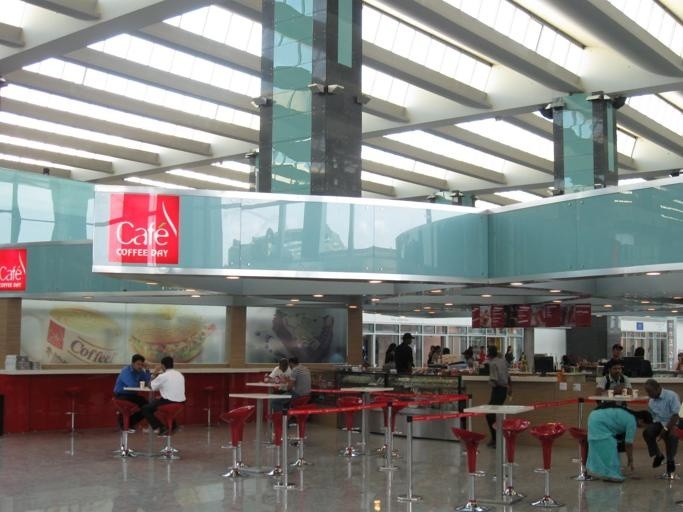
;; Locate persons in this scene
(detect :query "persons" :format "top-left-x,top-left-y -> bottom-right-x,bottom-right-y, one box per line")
385,343 -> 396,369
269,358 -> 296,412
430,343 -> 683,482
148,357 -> 186,435
396,334 -> 415,375
113,354 -> 150,433
287,357 -> 311,401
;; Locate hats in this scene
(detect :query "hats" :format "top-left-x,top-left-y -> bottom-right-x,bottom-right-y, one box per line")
613,344 -> 624,350
403,333 -> 415,339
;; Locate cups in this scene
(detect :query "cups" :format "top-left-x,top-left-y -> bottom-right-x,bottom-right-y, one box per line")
389,368 -> 397,374
139,380 -> 145,389
606,388 -> 638,399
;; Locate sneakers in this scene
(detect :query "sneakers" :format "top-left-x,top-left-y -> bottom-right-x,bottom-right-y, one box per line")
173,425 -> 183,433
154,428 -> 168,435
653,454 -> 665,467
487,442 -> 496,448
128,429 -> 136,434
667,459 -> 675,473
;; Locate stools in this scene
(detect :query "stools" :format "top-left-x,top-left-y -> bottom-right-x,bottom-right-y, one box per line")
65,385 -> 83,437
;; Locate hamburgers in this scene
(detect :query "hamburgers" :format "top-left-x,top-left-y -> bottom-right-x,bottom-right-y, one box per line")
130,304 -> 204,363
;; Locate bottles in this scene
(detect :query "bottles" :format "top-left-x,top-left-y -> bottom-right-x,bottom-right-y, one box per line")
557,367 -> 567,383
520,351 -> 525,360
263,372 -> 269,383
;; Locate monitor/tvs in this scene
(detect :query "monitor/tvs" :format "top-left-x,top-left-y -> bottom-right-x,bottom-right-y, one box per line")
535,357 -> 555,375
623,356 -> 646,377
